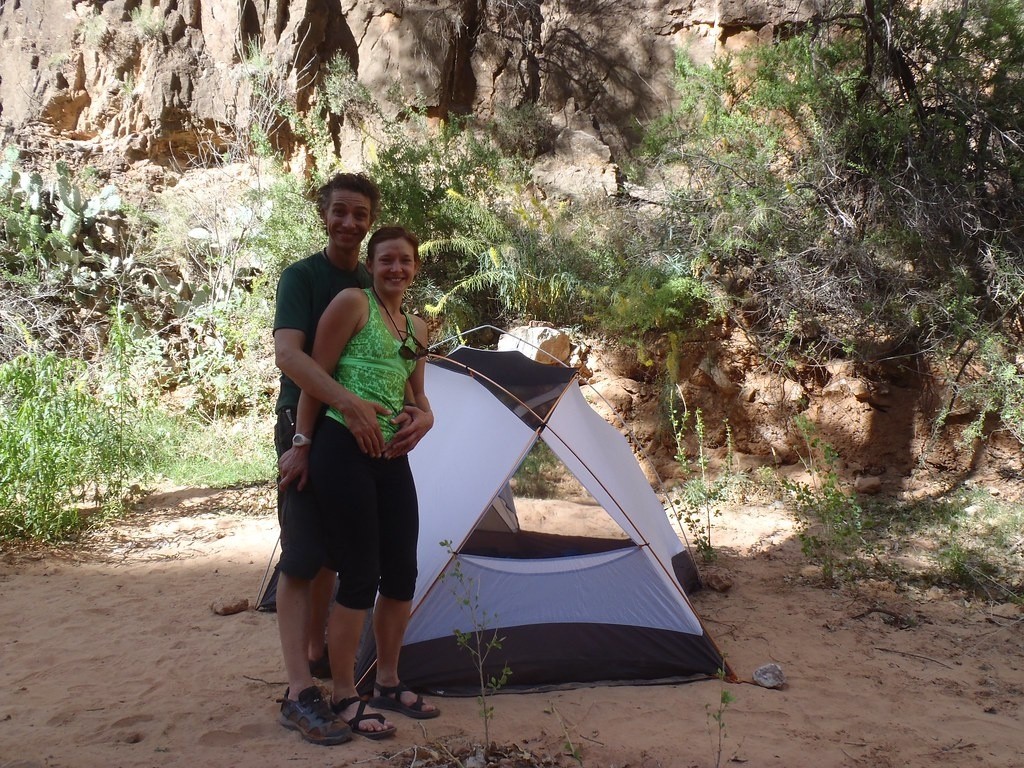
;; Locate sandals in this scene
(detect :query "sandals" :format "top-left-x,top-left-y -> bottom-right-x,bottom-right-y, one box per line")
369,682 -> 440,718
330,695 -> 397,739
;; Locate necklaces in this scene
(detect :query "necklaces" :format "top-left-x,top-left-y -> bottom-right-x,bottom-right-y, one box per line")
373,287 -> 410,342
324,247 -> 340,282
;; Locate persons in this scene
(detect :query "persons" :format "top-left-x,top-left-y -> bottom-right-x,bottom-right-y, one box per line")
273,172 -> 420,745
279,227 -> 440,739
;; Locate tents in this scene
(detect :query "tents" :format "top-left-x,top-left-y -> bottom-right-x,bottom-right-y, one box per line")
258,326 -> 741,695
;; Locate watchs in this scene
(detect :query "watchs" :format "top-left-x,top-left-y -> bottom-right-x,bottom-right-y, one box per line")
292,434 -> 311,446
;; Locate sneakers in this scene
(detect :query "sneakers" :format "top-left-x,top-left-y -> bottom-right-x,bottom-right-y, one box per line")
276,686 -> 352,746
308,645 -> 332,680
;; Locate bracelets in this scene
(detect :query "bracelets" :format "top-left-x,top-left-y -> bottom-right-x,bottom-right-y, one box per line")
405,403 -> 417,407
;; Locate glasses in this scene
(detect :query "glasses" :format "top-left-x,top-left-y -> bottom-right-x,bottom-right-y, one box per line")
399,330 -> 429,360
